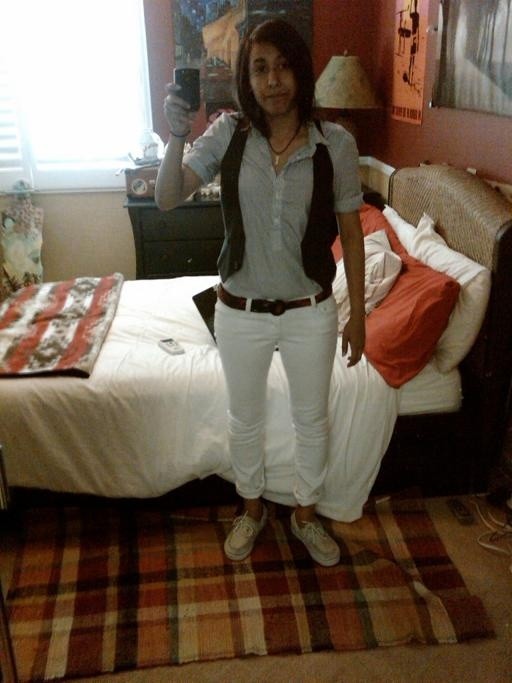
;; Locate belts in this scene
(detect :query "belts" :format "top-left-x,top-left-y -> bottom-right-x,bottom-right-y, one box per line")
215,284 -> 332,316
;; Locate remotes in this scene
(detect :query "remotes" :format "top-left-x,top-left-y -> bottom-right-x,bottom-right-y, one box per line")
447,497 -> 475,525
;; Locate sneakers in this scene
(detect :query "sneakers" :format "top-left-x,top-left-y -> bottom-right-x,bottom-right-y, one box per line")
224,504 -> 268,561
290,510 -> 341,567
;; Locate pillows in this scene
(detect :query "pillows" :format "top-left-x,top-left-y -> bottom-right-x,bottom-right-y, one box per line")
380,204 -> 416,254
329,201 -> 459,389
408,211 -> 492,375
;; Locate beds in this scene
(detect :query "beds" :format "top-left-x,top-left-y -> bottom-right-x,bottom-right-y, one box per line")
0,160 -> 512,519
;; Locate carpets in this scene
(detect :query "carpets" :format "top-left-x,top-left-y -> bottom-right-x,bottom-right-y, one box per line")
0,496 -> 496,683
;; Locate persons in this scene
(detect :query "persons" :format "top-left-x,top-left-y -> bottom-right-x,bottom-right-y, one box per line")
155,17 -> 366,568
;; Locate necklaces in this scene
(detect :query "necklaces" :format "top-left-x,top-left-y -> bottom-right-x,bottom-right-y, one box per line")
268,120 -> 303,166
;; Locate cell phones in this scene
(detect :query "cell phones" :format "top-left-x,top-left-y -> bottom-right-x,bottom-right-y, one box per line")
158,337 -> 184,355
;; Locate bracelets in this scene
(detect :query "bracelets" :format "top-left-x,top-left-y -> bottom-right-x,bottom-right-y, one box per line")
169,128 -> 192,138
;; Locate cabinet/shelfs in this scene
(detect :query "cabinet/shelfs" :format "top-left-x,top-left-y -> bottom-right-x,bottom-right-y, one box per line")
125,196 -> 226,280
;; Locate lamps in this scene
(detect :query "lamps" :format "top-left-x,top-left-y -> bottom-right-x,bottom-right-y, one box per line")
308,49 -> 387,135
